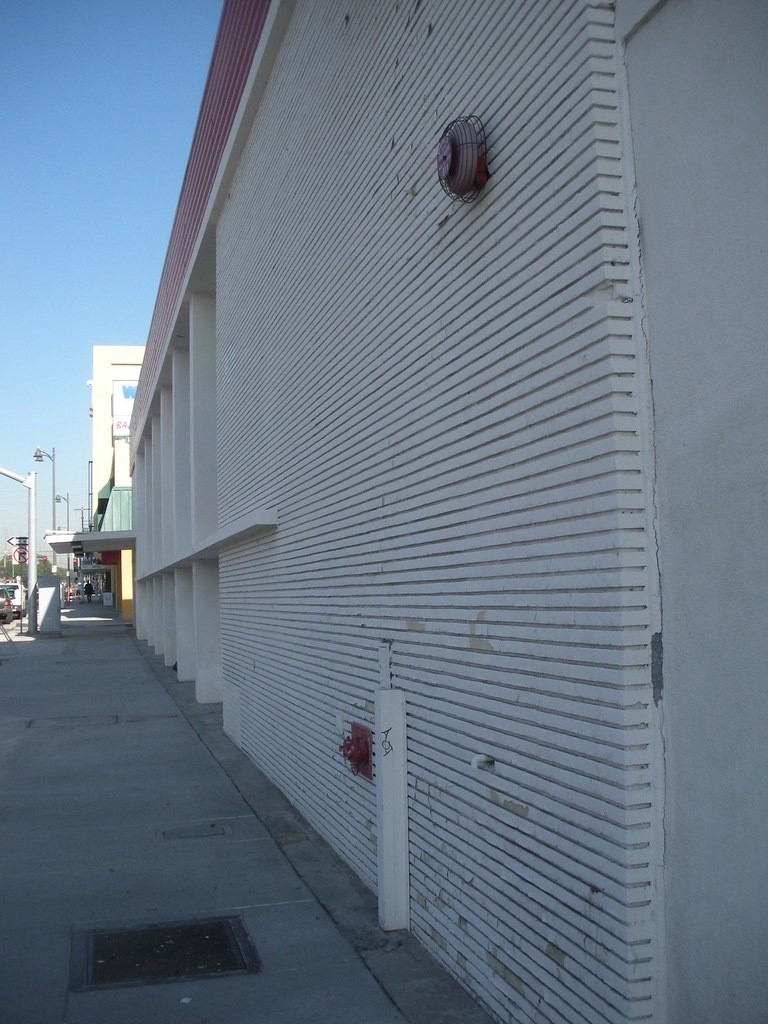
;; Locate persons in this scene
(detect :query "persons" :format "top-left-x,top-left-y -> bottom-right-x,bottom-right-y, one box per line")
84,580 -> 93,603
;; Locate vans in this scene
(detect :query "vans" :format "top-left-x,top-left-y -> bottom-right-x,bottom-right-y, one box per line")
0,581 -> 28,619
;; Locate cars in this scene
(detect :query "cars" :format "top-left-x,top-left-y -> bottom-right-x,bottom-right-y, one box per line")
0,587 -> 16,624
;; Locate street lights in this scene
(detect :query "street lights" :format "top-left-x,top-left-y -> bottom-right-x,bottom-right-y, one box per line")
33,446 -> 57,576
55,492 -> 70,603
